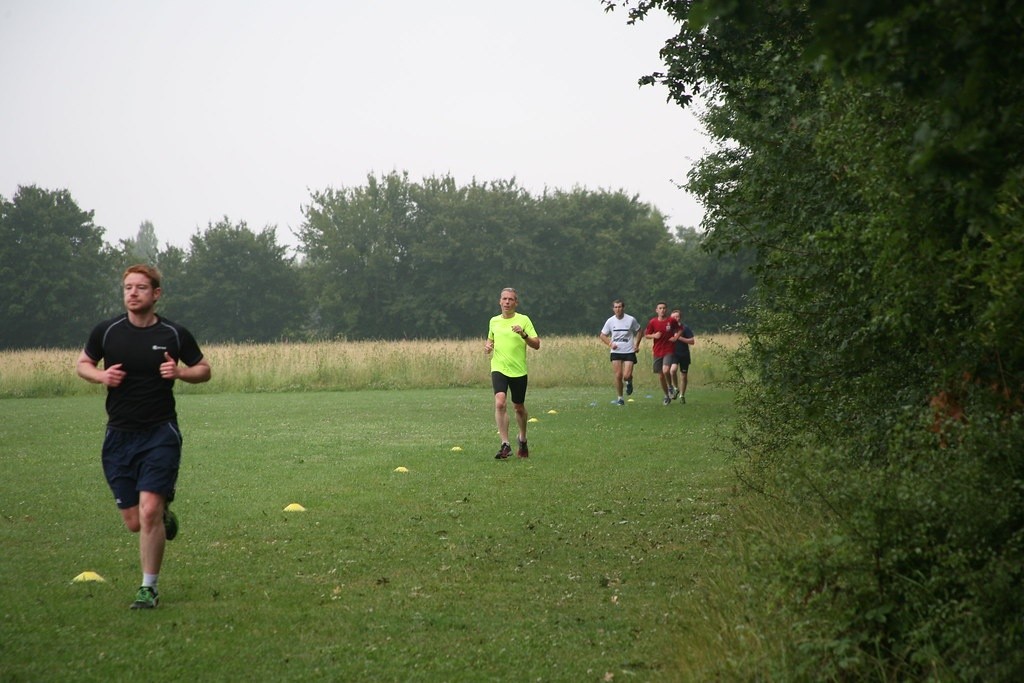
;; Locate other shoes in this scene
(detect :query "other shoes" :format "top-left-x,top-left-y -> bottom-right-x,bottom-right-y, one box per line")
625,378 -> 633,395
617,396 -> 624,405
664,386 -> 685,406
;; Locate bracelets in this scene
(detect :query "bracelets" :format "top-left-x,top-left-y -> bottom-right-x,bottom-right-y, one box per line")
521,333 -> 528,339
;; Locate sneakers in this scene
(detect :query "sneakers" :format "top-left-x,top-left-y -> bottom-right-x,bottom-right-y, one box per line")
163,502 -> 179,542
494,442 -> 513,460
516,434 -> 529,460
129,587 -> 159,609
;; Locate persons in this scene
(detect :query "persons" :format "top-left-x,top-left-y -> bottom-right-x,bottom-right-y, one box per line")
600,299 -> 643,406
644,302 -> 682,405
483,287 -> 543,461
76,261 -> 215,610
669,309 -> 694,404
